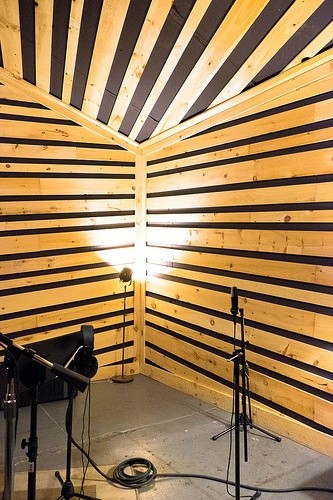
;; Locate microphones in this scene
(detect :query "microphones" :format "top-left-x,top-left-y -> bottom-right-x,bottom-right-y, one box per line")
19,359 -> 47,389
70,354 -> 98,379
230,287 -> 239,315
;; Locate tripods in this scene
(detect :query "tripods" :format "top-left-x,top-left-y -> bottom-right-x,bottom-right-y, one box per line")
213,307 -> 282,461
54,345 -> 101,500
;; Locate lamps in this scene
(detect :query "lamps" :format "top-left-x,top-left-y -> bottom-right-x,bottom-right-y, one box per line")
109,267 -> 134,383
